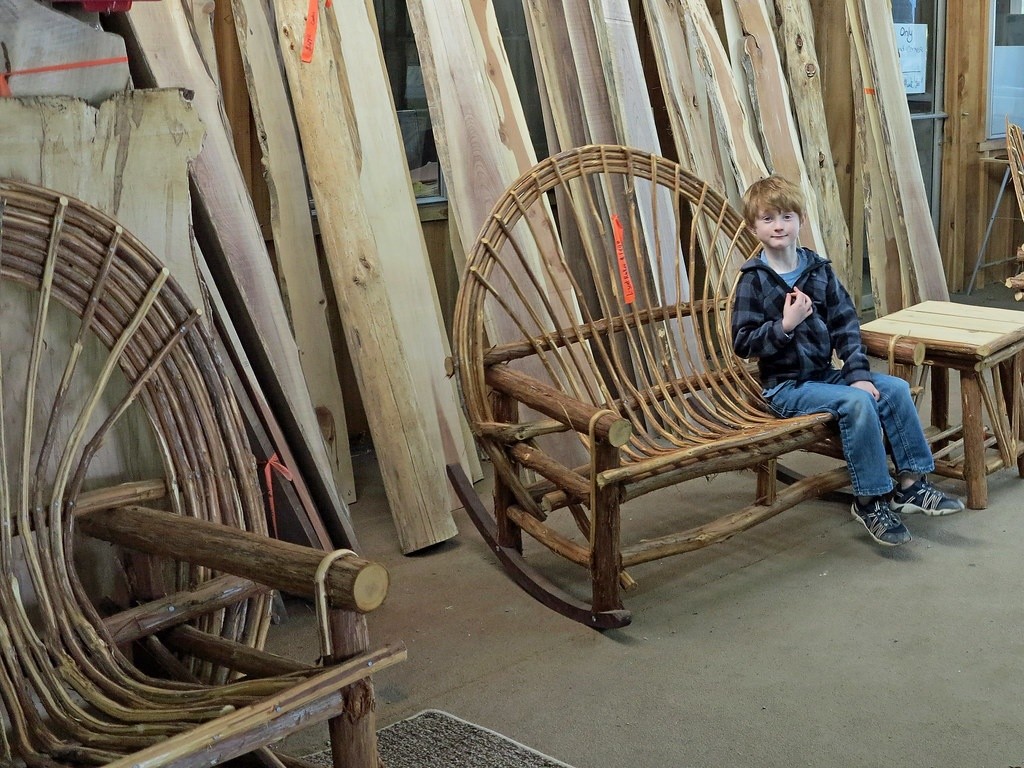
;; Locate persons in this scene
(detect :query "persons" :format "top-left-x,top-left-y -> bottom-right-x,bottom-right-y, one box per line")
732,176 -> 964,545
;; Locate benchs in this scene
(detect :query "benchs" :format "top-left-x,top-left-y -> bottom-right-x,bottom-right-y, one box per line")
443,143 -> 927,634
0,176 -> 410,768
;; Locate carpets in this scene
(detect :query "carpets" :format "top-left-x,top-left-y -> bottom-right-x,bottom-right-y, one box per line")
299,708 -> 574,768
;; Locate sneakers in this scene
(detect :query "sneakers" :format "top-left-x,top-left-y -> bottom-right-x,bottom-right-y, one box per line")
890,475 -> 965,517
850,495 -> 912,547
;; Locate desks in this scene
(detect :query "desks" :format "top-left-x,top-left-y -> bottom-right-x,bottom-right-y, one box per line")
861,298 -> 1024,508
